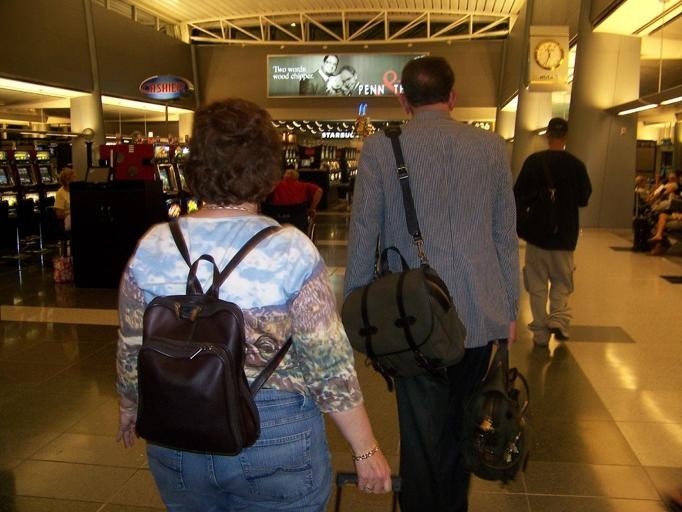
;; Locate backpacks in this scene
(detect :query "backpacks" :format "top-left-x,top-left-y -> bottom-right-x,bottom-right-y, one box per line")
133,217 -> 296,457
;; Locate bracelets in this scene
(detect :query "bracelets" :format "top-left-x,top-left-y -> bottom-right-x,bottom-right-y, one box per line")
350,445 -> 381,464
117,402 -> 138,416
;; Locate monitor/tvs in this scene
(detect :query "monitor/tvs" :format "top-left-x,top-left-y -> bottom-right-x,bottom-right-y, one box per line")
40,166 -> 52,183
159,167 -> 171,190
0,167 -> 10,186
179,168 -> 187,190
18,166 -> 32,184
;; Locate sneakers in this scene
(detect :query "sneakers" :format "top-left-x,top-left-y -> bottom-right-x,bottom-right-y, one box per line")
548,321 -> 568,341
533,331 -> 548,346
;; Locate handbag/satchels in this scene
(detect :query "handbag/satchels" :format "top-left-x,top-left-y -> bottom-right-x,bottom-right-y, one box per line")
341,123 -> 468,391
461,339 -> 532,486
53,256 -> 74,282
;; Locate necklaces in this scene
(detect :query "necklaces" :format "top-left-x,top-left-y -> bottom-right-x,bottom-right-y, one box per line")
199,203 -> 256,214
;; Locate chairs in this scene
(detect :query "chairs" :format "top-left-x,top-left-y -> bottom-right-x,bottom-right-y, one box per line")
3,195 -> 64,258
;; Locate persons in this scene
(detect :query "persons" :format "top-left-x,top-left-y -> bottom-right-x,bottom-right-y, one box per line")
299,53 -> 375,96
512,114 -> 594,347
111,93 -> 395,510
633,168 -> 680,256
339,51 -> 520,511
267,169 -> 324,220
53,167 -> 80,231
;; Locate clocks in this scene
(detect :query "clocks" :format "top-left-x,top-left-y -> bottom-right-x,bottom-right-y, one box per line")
535,39 -> 565,71
